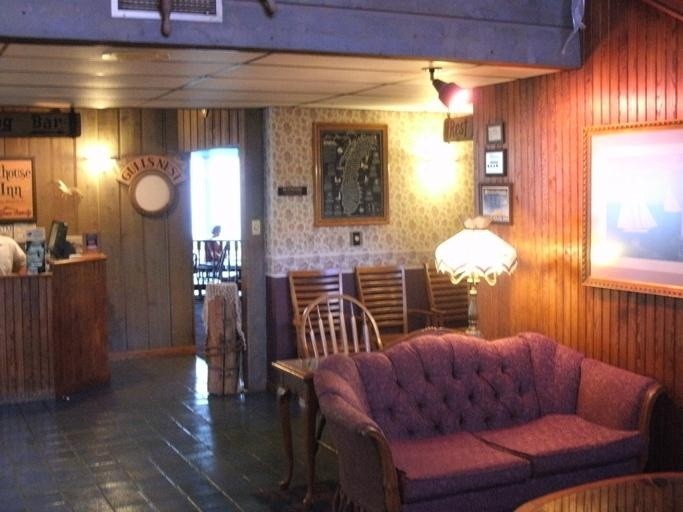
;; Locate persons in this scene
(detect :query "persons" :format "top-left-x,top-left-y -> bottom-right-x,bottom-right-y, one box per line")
0,235 -> 27,280
205,226 -> 227,262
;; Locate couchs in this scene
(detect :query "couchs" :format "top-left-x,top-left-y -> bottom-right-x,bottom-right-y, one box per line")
314,332 -> 667,511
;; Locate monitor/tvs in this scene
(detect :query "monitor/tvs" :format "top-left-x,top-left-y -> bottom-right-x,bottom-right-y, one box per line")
47,219 -> 68,259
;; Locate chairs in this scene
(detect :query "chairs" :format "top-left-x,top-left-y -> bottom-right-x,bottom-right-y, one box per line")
299,293 -> 386,510
287,269 -> 367,360
423,260 -> 472,334
353,264 -> 434,349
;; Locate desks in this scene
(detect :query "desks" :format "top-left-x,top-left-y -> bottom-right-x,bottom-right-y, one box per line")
270,356 -> 340,508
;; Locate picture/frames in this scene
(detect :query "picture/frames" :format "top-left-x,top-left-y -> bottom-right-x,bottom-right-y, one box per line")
484,148 -> 507,176
479,182 -> 512,226
486,121 -> 506,145
313,121 -> 390,227
0,157 -> 37,224
579,119 -> 683,299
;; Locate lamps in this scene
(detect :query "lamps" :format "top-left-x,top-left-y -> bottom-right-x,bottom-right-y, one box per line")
430,67 -> 469,115
435,217 -> 517,338
